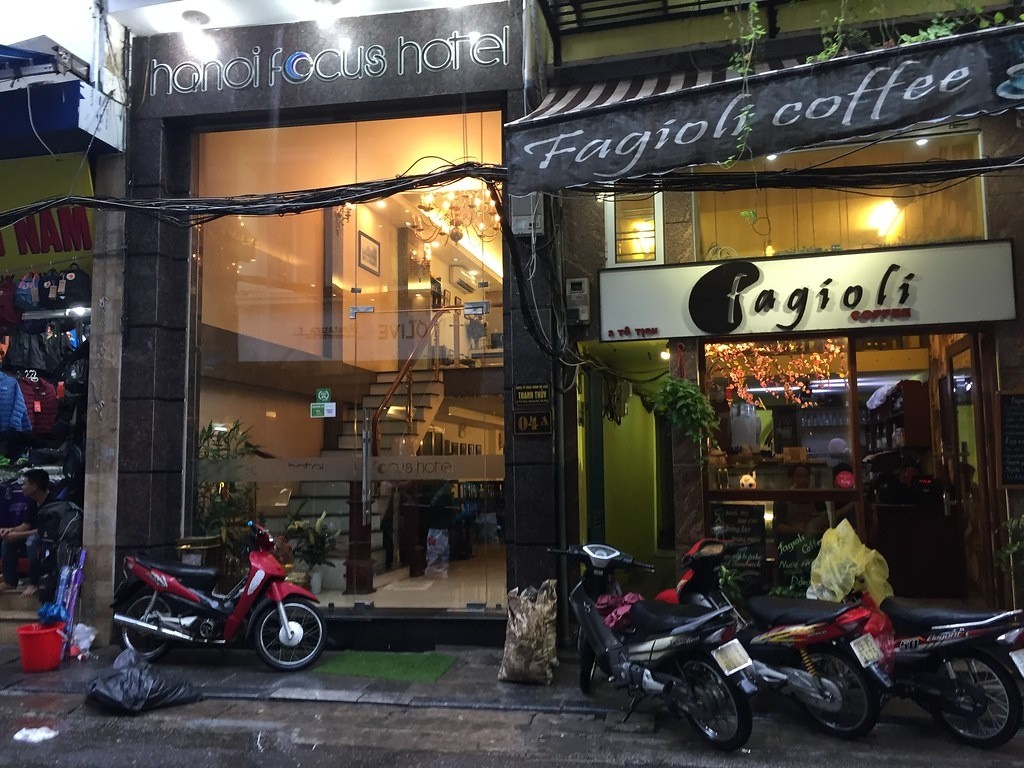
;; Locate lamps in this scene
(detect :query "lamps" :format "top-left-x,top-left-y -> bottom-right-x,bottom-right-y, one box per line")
410,249 -> 432,284
403,112 -> 503,247
891,187 -> 916,209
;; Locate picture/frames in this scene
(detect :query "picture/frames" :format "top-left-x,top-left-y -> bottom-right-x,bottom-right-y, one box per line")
358,230 -> 381,278
452,442 -> 482,455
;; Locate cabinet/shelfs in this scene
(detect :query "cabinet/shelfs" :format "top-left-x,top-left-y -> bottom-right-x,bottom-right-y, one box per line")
864,380 -> 931,452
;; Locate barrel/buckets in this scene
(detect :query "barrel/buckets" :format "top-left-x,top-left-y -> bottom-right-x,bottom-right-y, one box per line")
17,621 -> 69,673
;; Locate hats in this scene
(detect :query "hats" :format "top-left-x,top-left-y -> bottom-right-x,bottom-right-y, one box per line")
828,438 -> 848,454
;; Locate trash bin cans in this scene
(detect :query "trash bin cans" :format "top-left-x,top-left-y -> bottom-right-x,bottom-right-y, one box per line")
15,623 -> 67,675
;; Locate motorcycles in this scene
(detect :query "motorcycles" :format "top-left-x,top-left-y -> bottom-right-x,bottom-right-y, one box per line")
113,520 -> 328,670
654,526 -> 894,740
541,542 -> 753,753
807,517 -> 1023,750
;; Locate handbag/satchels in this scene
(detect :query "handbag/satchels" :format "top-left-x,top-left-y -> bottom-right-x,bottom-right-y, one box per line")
58,263 -> 91,307
14,272 -> 40,310
36,501 -> 84,547
424,528 -> 449,579
0,274 -> 22,323
38,268 -> 71,309
497,578 -> 559,685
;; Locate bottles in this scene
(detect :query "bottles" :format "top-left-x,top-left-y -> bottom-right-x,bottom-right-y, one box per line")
801,407 -> 897,451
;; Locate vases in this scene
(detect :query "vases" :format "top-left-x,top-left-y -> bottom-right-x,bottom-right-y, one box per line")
310,568 -> 323,594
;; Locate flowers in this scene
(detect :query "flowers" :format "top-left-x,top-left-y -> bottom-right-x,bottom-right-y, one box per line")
287,510 -> 342,571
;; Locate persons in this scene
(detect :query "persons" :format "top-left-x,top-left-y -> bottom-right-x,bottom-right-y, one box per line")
0,469 -> 64,600
771,461 -> 829,570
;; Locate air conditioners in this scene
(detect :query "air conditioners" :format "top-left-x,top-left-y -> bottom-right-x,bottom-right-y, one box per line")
448,264 -> 476,294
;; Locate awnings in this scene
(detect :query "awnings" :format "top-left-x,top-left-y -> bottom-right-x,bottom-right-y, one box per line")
499,20 -> 1024,194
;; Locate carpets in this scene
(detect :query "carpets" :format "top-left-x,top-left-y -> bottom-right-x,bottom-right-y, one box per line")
313,649 -> 460,685
